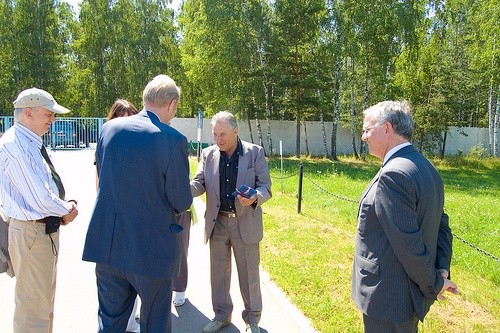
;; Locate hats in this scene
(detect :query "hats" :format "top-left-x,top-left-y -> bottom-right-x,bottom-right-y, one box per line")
13,87 -> 71,114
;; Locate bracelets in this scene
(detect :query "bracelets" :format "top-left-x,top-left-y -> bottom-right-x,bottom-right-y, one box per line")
67,199 -> 77,205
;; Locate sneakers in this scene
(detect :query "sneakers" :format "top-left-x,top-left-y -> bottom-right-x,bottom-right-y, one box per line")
245,323 -> 261,333
127,323 -> 140,333
203,320 -> 230,333
172,291 -> 185,306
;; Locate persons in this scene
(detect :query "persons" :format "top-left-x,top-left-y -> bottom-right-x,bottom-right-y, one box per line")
81,74 -> 194,333
350,100 -> 461,333
96,99 -> 140,333
173,205 -> 197,305
0,87 -> 78,333
190,111 -> 273,333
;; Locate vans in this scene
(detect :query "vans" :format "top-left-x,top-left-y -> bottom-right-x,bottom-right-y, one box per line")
49,120 -> 87,147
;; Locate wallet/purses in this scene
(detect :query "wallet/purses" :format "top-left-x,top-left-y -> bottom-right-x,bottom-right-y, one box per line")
232,184 -> 258,199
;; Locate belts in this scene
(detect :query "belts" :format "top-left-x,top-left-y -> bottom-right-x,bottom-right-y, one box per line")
218,211 -> 237,218
29,216 -> 63,224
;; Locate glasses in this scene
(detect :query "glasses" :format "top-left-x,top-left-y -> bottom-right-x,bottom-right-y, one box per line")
362,122 -> 385,133
